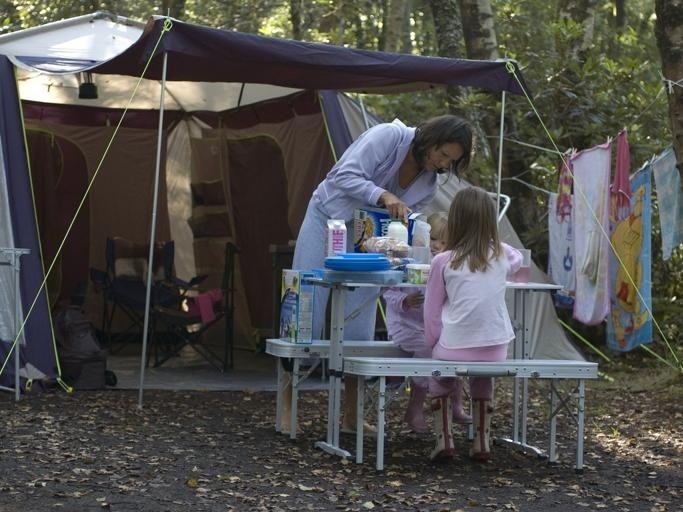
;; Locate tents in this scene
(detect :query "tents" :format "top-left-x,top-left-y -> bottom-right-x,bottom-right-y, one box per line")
1,11 -> 533,397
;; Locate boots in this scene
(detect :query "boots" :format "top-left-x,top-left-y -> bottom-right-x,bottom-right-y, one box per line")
341,376 -> 386,437
280,373 -> 303,435
469,398 -> 493,460
440,377 -> 472,424
430,395 -> 454,461
404,381 -> 429,434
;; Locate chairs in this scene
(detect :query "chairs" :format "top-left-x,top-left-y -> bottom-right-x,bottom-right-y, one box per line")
146,242 -> 240,374
89,236 -> 181,369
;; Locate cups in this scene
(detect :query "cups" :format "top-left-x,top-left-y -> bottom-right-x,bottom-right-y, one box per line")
514,246 -> 531,284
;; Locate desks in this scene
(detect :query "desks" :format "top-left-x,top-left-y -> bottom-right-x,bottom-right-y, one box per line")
300,279 -> 569,457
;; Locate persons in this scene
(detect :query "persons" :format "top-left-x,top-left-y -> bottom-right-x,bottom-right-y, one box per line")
383,210 -> 473,435
422,186 -> 525,462
274,113 -> 474,435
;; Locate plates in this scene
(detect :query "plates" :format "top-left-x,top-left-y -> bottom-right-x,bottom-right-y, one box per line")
322,250 -> 393,273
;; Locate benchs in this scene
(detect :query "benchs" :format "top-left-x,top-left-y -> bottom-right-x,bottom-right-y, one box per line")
342,355 -> 600,474
264,338 -> 416,446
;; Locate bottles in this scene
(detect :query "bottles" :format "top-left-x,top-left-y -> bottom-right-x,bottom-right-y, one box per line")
404,263 -> 431,284
386,220 -> 407,244
